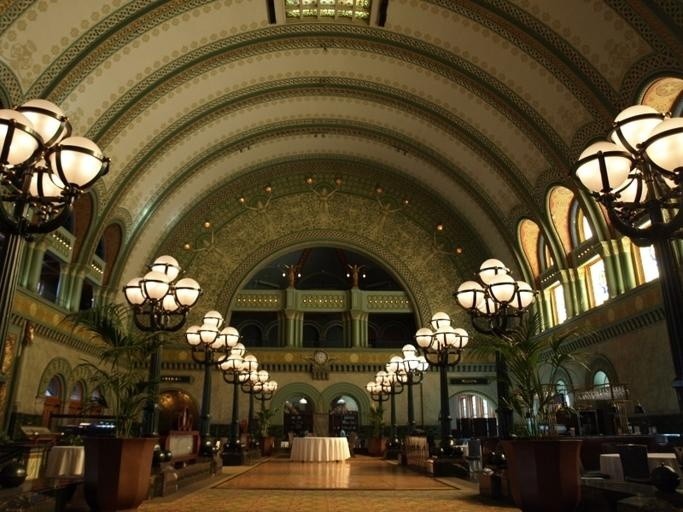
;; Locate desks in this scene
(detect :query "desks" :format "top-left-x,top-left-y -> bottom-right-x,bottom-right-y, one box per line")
597,451 -> 681,483
291,435 -> 352,464
44,444 -> 88,475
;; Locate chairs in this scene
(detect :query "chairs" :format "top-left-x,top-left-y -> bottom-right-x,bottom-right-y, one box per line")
580,435 -> 652,485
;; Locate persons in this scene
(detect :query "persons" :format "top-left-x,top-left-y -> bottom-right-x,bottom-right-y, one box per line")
309,184 -> 339,224
243,192 -> 279,235
189,229 -> 232,274
347,261 -> 361,286
421,229 -> 453,268
284,262 -> 297,286
369,193 -> 404,232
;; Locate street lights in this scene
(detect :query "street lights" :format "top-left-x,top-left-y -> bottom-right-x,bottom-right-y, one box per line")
364,312 -> 469,450
0,99 -> 110,375
123,255 -> 202,436
184,310 -> 279,442
569,106 -> 682,411
454,259 -> 540,440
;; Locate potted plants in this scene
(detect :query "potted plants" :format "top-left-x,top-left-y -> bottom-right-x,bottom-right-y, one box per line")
364,406 -> 386,455
51,297 -> 172,511
455,303 -> 588,510
255,407 -> 281,457
